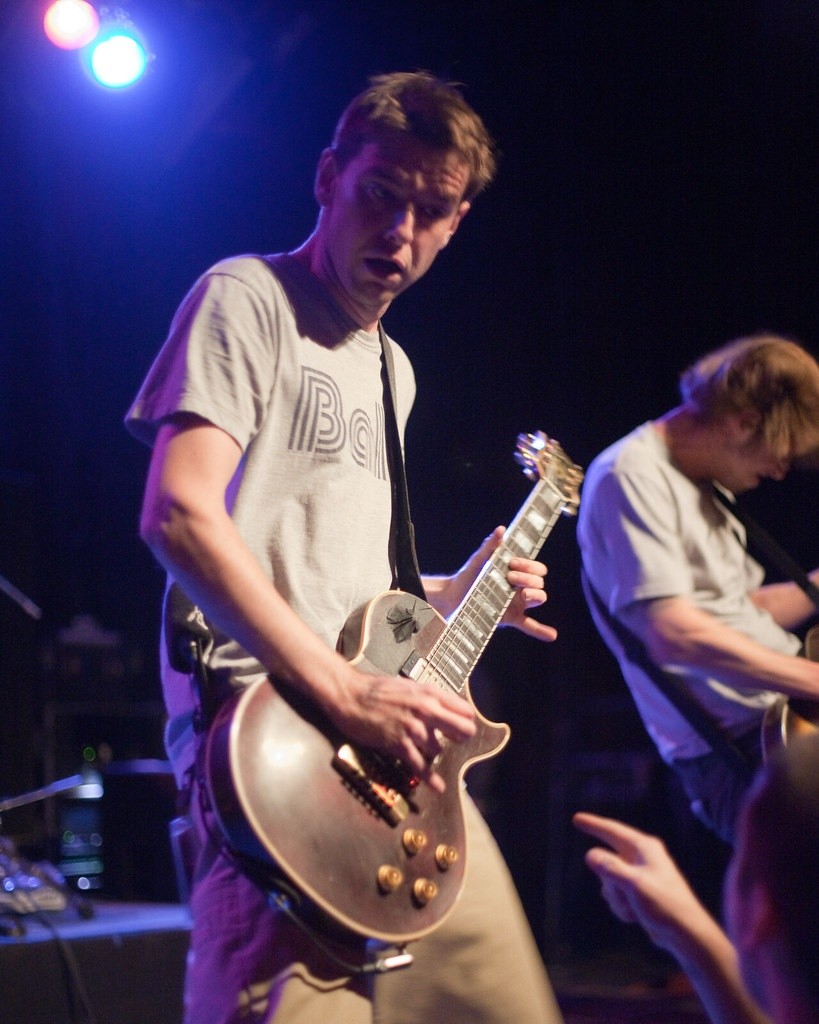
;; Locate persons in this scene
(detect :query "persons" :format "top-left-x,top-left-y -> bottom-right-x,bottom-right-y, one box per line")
125,76 -> 557,1024
572,726 -> 819,1024
577,333 -> 819,848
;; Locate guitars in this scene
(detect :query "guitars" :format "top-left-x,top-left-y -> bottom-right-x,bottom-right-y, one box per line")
759,624 -> 819,817
197,432 -> 590,968
